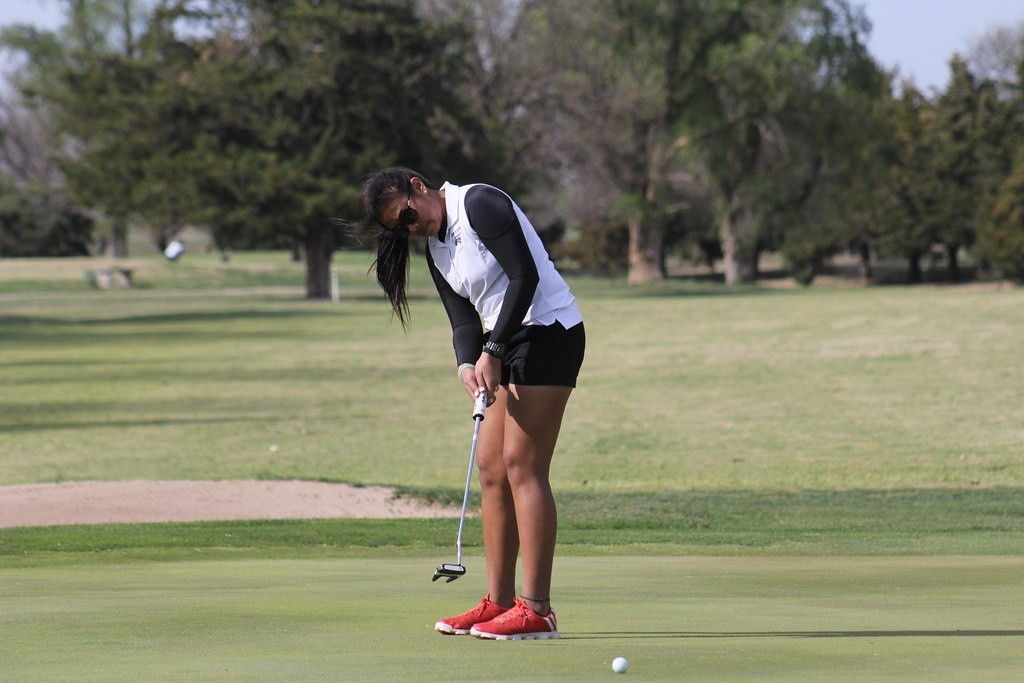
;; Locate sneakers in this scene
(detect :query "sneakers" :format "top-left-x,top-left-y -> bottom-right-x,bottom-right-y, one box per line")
434,593 -> 518,635
470,595 -> 561,641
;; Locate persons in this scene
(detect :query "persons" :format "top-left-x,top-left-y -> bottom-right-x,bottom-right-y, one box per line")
364,167 -> 587,641
164,239 -> 185,262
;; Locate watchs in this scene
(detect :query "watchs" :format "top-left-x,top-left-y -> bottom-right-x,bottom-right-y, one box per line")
486,342 -> 505,356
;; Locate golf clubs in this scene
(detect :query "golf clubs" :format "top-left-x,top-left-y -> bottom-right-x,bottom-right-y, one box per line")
432,389 -> 488,583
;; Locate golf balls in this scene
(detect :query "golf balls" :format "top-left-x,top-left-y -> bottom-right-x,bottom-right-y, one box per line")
611,656 -> 628,673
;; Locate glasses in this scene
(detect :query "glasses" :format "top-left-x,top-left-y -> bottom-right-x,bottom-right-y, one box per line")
392,189 -> 418,237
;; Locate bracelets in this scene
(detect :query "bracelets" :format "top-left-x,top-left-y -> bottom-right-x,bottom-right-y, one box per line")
457,363 -> 474,384
